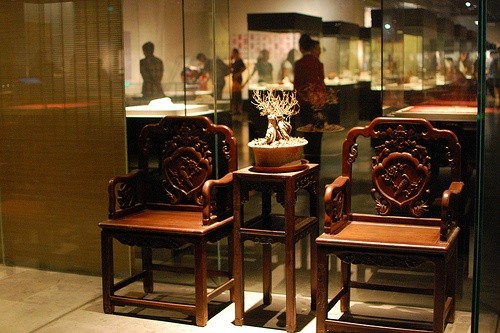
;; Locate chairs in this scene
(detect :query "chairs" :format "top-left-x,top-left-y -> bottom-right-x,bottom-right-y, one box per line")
315,116 -> 465,333
97,115 -> 238,327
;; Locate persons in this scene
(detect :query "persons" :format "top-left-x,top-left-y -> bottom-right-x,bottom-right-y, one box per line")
139,41 -> 164,99
437,46 -> 500,98
180,37 -> 326,171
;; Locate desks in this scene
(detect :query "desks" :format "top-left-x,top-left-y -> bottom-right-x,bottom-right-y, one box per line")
232,164 -> 319,333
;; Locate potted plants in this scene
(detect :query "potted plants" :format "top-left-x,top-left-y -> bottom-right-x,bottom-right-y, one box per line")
247,86 -> 309,171
